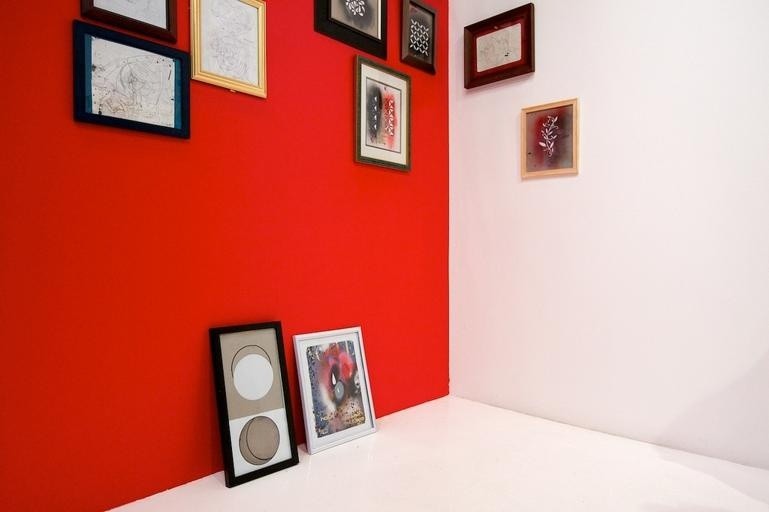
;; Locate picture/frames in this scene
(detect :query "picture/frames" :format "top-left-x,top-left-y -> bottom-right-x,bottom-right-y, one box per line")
520,96 -> 578,178
463,3 -> 537,89
72,18 -> 191,140
399,0 -> 438,76
292,326 -> 378,455
80,0 -> 178,43
207,321 -> 300,488
352,53 -> 413,174
189,0 -> 270,102
313,0 -> 388,60
